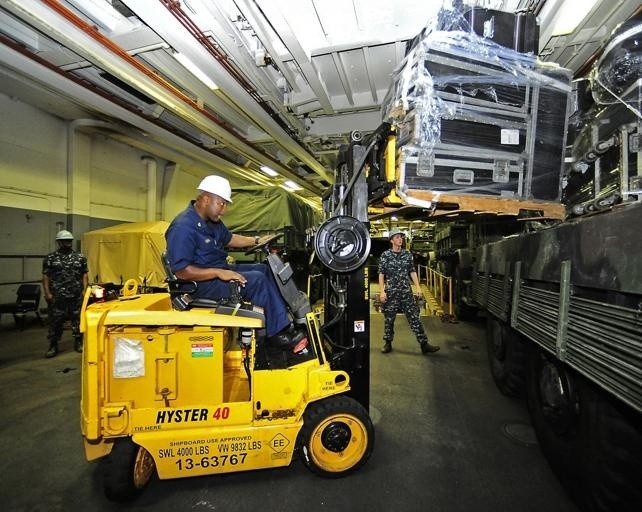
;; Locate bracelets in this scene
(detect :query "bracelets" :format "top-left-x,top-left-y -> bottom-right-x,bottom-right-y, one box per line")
254,236 -> 261,246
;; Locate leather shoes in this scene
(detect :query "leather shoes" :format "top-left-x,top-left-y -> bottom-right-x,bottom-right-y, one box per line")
264,328 -> 305,352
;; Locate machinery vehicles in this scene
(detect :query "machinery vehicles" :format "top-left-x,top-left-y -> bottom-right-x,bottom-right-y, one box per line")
73,119 -> 528,507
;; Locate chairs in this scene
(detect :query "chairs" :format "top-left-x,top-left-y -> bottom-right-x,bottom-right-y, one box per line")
0,284 -> 43,332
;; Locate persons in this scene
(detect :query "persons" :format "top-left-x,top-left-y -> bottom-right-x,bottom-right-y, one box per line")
41,227 -> 91,360
375,226 -> 441,355
160,174 -> 308,352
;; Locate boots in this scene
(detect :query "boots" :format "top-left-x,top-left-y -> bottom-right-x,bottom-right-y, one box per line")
421,341 -> 440,353
381,340 -> 391,353
74,338 -> 82,353
45,341 -> 57,358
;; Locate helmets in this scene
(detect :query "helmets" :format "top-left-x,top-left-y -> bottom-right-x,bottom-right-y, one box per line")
55,229 -> 74,241
195,174 -> 234,204
389,228 -> 406,239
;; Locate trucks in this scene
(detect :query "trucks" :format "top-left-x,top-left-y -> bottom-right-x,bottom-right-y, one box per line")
427,199 -> 642,512
216,185 -> 327,307
